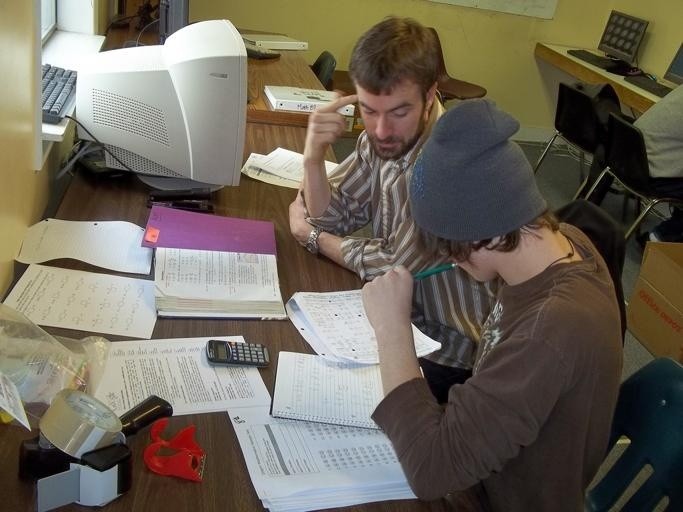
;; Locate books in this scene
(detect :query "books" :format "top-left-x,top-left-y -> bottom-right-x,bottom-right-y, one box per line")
244,32 -> 312,52
264,82 -> 358,115
142,201 -> 287,323
271,350 -> 424,429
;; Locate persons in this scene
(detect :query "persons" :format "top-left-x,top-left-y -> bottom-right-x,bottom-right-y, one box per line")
357,94 -> 625,512
289,15 -> 501,403
630,79 -> 683,252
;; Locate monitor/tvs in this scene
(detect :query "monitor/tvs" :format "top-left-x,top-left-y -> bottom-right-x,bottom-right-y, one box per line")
597,10 -> 649,64
122,0 -> 188,49
76,18 -> 247,197
662,41 -> 683,86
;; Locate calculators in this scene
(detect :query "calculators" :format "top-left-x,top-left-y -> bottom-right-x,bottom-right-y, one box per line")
205,340 -> 270,366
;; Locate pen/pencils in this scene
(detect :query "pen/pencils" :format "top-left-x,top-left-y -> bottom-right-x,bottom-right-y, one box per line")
413,263 -> 458,280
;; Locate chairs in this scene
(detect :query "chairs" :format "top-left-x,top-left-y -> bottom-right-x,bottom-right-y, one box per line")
554,199 -> 627,346
583,358 -> 683,512
427,27 -> 487,106
311,51 -> 336,88
583,112 -> 683,239
534,82 -> 635,202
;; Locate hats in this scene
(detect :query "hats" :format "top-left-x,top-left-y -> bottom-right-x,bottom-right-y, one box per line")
406,98 -> 546,241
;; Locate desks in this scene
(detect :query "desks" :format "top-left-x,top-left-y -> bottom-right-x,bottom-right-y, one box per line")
99,25 -> 354,132
0,122 -> 492,512
535,42 -> 674,120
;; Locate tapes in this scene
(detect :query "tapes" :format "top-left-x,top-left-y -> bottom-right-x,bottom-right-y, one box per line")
37,389 -> 123,459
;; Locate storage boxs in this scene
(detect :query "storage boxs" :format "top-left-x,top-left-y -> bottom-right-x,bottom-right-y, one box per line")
624,241 -> 683,366
309,65 -> 365,138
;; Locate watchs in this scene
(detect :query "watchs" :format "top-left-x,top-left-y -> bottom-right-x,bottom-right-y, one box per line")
306,226 -> 327,253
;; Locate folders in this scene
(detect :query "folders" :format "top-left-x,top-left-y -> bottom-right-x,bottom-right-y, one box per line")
263,85 -> 355,117
241,34 -> 310,51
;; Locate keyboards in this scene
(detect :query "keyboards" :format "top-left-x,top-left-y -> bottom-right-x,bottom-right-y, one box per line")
244,41 -> 281,59
624,74 -> 674,99
41,62 -> 77,125
567,50 -> 613,71
